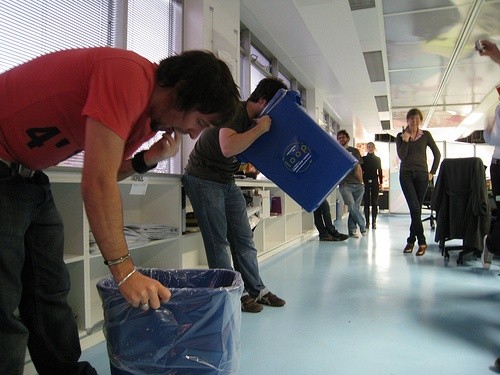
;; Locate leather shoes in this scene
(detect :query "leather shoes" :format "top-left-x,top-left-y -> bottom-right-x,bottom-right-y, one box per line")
403,243 -> 414,253
416,244 -> 427,256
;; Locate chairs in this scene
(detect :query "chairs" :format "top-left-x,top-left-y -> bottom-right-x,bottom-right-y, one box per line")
420,177 -> 437,230
431,156 -> 491,264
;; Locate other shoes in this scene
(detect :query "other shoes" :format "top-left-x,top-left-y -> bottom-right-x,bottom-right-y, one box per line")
365,222 -> 370,229
372,222 -> 376,229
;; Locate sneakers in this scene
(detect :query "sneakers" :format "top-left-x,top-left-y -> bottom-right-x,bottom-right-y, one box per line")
361,224 -> 366,235
481,234 -> 494,270
332,231 -> 349,240
240,295 -> 263,313
349,231 -> 358,238
321,234 -> 339,241
258,291 -> 286,306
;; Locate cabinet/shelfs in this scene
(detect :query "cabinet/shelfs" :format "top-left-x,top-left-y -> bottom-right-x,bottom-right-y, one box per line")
22,171 -> 344,375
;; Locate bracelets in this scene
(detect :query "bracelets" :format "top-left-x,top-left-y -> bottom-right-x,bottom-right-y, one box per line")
118,266 -> 136,287
103,253 -> 130,266
133,150 -> 159,175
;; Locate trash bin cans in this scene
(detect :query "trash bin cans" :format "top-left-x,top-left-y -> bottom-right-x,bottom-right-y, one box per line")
236,88 -> 358,212
97,266 -> 244,375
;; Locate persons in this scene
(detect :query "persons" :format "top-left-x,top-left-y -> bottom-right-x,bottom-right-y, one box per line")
336,129 -> 368,238
396,108 -> 441,256
183,76 -> 286,311
476,39 -> 500,67
313,200 -> 349,242
482,104 -> 500,274
0,46 -> 242,375
361,142 -> 383,231
